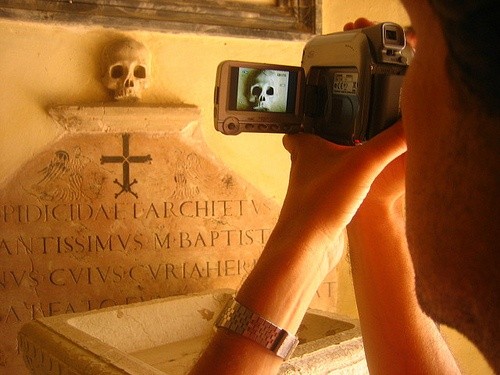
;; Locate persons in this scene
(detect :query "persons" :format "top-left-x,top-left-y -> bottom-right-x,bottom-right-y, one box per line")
184,0 -> 499,375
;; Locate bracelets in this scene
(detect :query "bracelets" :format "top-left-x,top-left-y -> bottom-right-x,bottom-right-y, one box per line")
211,292 -> 300,362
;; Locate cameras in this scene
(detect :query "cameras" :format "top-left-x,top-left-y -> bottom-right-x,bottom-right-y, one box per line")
213,21 -> 414,148
